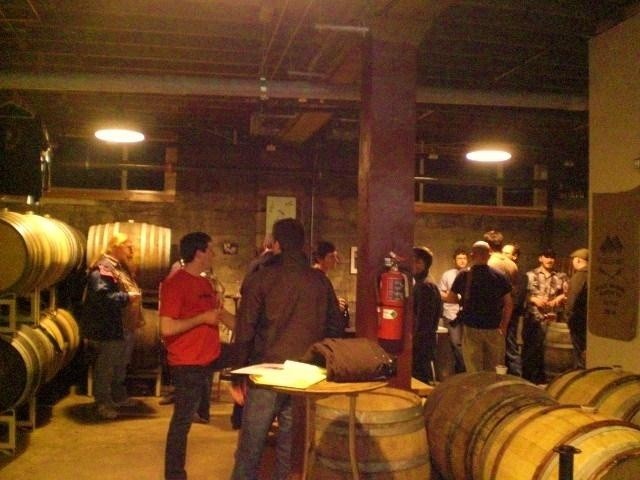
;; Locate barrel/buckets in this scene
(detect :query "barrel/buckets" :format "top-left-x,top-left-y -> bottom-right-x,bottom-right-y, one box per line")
0,206 -> 86,413
545,366 -> 640,424
543,322 -> 575,378
132,308 -> 160,369
86,220 -> 172,286
314,387 -> 432,480
423,370 -> 558,480
480,402 -> 640,480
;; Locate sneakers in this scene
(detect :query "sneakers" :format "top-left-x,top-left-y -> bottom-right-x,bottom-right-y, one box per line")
95,392 -> 176,420
190,412 -> 210,424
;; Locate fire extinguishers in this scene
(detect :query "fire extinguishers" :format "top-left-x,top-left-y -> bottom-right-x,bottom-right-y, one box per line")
377,252 -> 409,342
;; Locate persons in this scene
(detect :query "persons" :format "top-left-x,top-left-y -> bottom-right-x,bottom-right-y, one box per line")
159,231 -> 236,480
412,246 -> 442,385
439,245 -> 467,374
78,233 -> 146,421
561,248 -> 588,371
232,218 -> 344,480
448,241 -> 513,372
521,247 -> 570,383
483,230 -> 528,375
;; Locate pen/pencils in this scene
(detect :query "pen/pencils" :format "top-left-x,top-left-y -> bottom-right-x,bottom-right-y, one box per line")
262,365 -> 285,370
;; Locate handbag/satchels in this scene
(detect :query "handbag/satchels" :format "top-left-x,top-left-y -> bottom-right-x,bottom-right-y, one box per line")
448,309 -> 462,350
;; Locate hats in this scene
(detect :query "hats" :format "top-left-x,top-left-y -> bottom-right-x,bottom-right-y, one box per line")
539,246 -> 589,259
